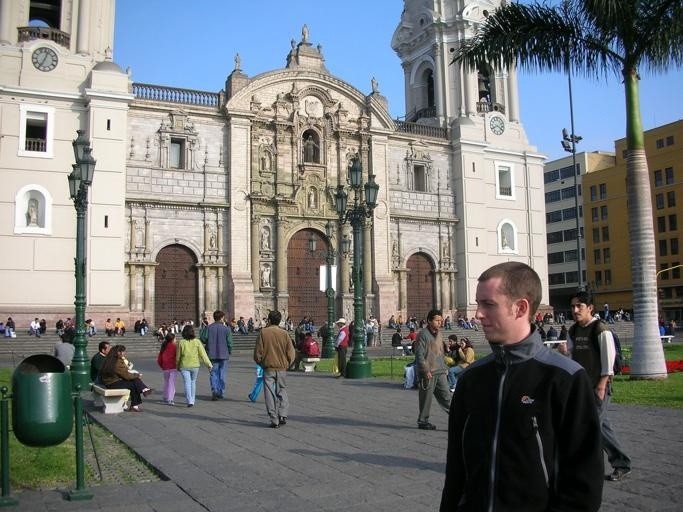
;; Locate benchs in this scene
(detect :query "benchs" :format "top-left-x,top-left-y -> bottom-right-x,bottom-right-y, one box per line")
543,340 -> 567,350
301,358 -> 320,373
394,346 -> 413,356
89,382 -> 129,415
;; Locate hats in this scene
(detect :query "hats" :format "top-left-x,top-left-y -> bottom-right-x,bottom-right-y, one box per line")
336,318 -> 346,323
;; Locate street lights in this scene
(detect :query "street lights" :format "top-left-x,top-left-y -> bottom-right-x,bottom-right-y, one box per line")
67,130 -> 96,391
333,156 -> 380,379
308,220 -> 350,359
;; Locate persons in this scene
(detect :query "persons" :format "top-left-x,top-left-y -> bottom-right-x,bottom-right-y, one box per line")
433,260 -> 603,512
559,291 -> 632,480
262,268 -> 271,286
261,229 -> 270,249
658,310 -> 677,336
366,307 -> 480,431
308,189 -> 315,206
303,135 -> 320,163
0,315 -> 74,338
27,201 -> 38,225
260,151 -> 271,170
533,311 -> 568,350
84,310 -> 355,428
593,300 -> 631,325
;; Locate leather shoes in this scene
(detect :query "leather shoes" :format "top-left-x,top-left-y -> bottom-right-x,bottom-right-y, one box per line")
143,388 -> 155,397
130,405 -> 143,412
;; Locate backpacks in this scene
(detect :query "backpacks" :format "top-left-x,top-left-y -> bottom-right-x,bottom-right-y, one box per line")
591,320 -> 624,374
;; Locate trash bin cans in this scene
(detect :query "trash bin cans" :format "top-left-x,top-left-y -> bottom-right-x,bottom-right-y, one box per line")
12,353 -> 74,447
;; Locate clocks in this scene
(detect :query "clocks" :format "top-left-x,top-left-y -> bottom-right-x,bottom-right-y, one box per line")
490,116 -> 504,135
32,47 -> 58,72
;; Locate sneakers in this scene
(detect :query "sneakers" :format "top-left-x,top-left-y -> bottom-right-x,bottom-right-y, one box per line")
272,417 -> 286,427
418,422 -> 436,430
609,467 -> 631,481
